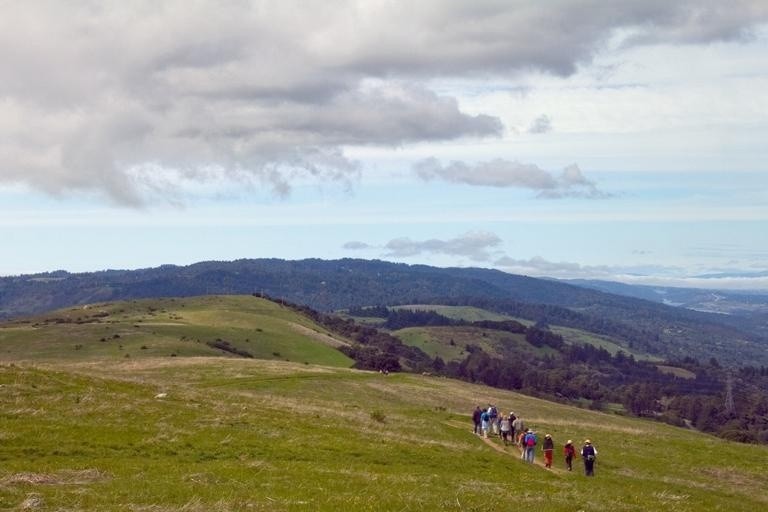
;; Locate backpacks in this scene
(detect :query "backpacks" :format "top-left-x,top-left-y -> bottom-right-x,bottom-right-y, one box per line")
491,407 -> 497,418
483,414 -> 489,421
527,436 -> 535,447
522,434 -> 527,446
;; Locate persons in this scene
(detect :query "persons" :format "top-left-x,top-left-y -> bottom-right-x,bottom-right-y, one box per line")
579,439 -> 598,476
471,402 -> 538,464
562,439 -> 577,471
543,433 -> 553,468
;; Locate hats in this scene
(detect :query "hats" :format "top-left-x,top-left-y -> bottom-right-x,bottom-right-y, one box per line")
546,434 -> 552,439
585,440 -> 591,444
567,439 -> 573,444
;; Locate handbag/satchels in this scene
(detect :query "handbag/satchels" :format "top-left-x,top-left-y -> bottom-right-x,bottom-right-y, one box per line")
588,454 -> 594,461
